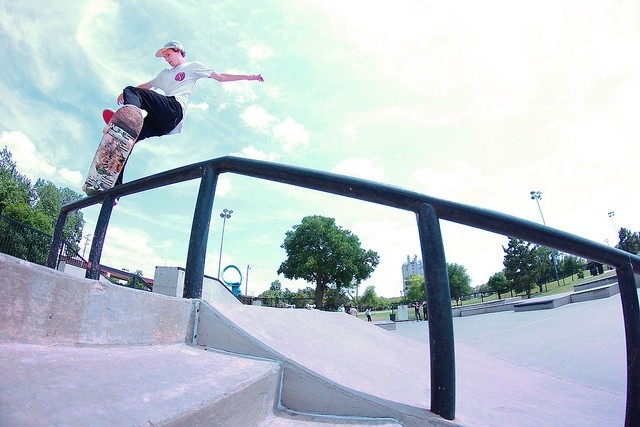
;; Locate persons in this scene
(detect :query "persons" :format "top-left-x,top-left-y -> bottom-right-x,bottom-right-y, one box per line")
85,39 -> 266,206
411,300 -> 423,321
422,301 -> 429,320
337,302 -> 346,312
365,306 -> 372,321
346,306 -> 358,317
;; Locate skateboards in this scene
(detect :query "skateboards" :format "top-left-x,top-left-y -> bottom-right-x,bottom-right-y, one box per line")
82,105 -> 143,204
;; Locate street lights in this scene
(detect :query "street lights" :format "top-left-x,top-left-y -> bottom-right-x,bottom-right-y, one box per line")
218,208 -> 233,279
530,190 -> 558,265
607,211 -> 621,243
245,264 -> 251,296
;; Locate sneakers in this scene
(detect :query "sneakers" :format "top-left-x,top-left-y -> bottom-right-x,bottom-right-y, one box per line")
102,108 -> 114,124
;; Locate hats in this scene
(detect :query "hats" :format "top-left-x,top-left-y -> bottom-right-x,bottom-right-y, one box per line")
155,40 -> 184,57
